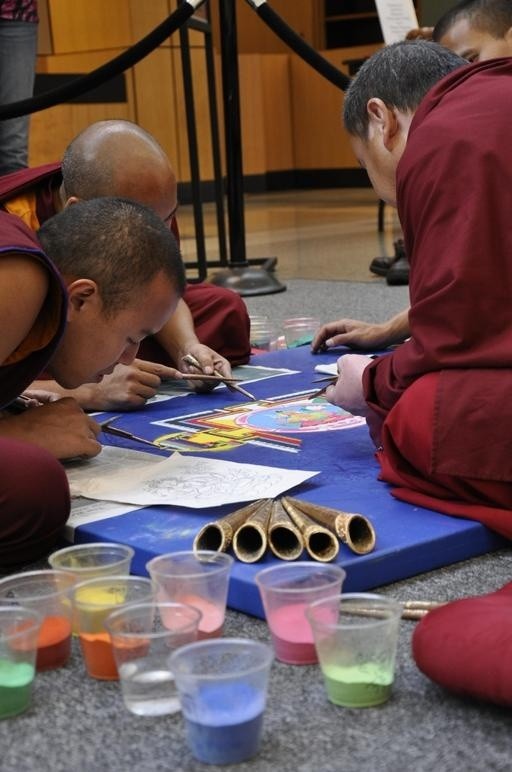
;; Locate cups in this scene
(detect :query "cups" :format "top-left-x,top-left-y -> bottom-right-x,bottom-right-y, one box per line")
64,575 -> 160,681
147,549 -> 235,649
104,603 -> 204,717
250,317 -> 320,354
252,559 -> 346,665
306,592 -> 404,709
48,542 -> 136,637
168,639 -> 277,765
0,569 -> 78,672
1,606 -> 43,720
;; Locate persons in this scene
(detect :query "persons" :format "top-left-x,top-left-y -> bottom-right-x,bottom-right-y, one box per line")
326,41 -> 512,539
0,120 -> 250,411
0,197 -> 186,578
310,1 -> 511,354
0,0 -> 40,174
